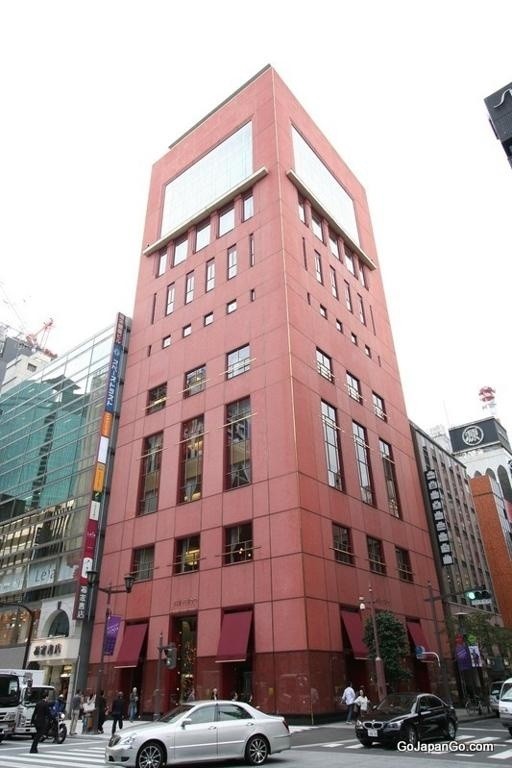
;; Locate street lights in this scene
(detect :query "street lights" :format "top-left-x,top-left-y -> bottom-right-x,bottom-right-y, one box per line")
86,570 -> 137,735
358,584 -> 387,702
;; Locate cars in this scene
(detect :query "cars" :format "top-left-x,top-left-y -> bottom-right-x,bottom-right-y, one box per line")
354,690 -> 459,750
488,681 -> 505,718
498,678 -> 512,736
104,699 -> 292,768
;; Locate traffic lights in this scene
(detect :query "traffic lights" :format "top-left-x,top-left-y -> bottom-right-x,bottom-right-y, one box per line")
164,647 -> 178,670
467,589 -> 492,601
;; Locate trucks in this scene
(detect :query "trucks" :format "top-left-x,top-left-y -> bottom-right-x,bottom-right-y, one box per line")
0,668 -> 57,743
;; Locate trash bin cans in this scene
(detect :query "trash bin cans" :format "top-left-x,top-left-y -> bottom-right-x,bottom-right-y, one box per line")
83,709 -> 93,734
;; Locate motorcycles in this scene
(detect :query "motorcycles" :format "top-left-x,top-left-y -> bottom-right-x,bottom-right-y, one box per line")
38,711 -> 68,744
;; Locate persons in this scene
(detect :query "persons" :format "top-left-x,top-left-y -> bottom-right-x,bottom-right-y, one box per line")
54,693 -> 68,714
110,691 -> 126,734
210,688 -> 219,700
352,689 -> 370,720
470,649 -> 480,667
233,691 -> 241,702
83,691 -> 96,727
129,687 -> 139,725
186,688 -> 196,702
352,685 -> 365,721
246,691 -> 253,704
339,680 -> 358,725
66,688 -> 86,736
29,691 -> 53,754
93,688 -> 107,734
